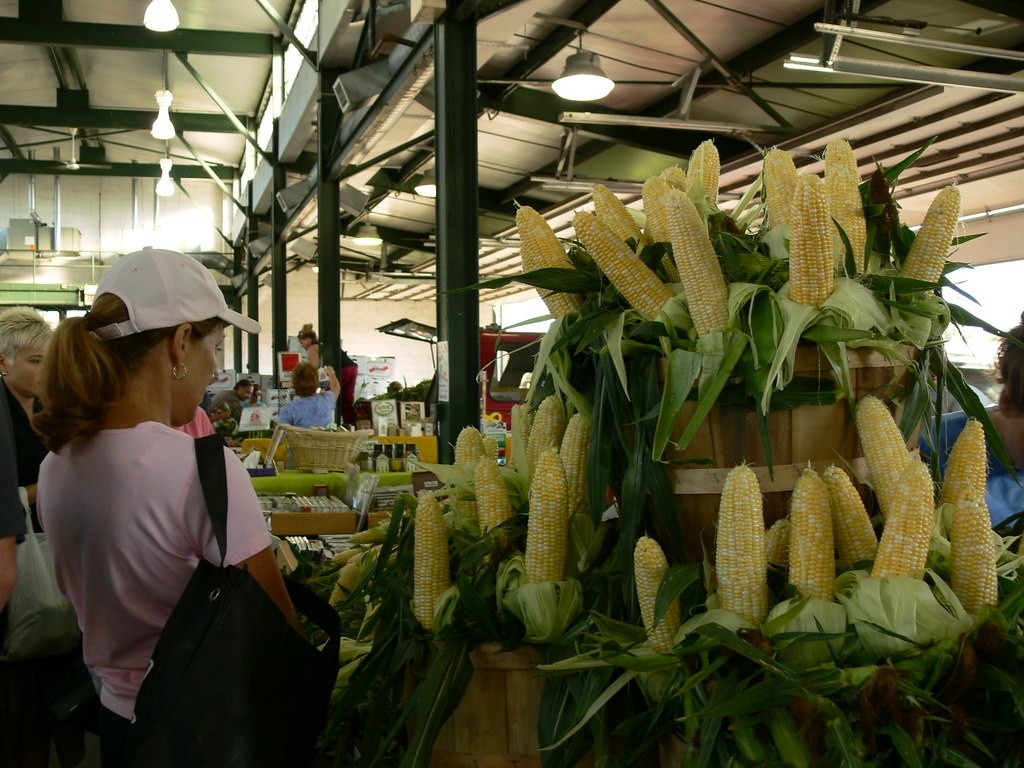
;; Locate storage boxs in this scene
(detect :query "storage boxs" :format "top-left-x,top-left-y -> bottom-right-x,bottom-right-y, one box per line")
387,422 -> 423,437
199,354 -> 395,419
412,471 -> 449,503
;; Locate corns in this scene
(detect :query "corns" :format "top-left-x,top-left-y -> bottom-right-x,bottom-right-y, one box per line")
328,394 -> 998,706
514,139 -> 961,339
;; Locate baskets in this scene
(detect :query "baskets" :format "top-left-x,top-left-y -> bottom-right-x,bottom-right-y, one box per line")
278,422 -> 375,472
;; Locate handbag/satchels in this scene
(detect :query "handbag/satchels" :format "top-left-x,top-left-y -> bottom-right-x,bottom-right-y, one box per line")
6,487 -> 82,660
126,430 -> 340,768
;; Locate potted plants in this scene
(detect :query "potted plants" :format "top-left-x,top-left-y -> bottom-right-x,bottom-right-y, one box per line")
421,417 -> 435,436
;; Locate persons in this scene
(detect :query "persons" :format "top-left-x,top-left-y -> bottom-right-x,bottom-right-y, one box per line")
243,383 -> 266,409
0,380 -> 27,613
0,307 -> 55,534
921,325 -> 1024,542
30,246 -> 300,768
171,406 -> 215,439
211,379 -> 254,422
298,324 -> 358,427
276,360 -> 341,430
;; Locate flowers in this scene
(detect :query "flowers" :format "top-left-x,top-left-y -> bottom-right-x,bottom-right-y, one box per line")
356,407 -> 369,419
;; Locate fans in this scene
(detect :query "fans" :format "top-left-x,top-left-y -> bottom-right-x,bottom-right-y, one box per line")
48,128 -> 112,170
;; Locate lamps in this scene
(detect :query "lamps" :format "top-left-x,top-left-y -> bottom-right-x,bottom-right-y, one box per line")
414,165 -> 437,198
551,49 -> 615,102
783,51 -> 1024,96
353,221 -> 383,246
313,260 -> 318,272
150,90 -> 175,140
155,158 -> 175,197
143,0 -> 180,32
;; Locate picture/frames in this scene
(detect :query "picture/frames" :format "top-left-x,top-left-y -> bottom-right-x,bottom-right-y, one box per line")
399,401 -> 426,430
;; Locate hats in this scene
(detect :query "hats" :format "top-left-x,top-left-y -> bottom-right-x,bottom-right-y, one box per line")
82,246 -> 262,336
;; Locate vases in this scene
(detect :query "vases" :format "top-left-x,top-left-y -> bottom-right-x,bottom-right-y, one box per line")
357,419 -> 371,430
